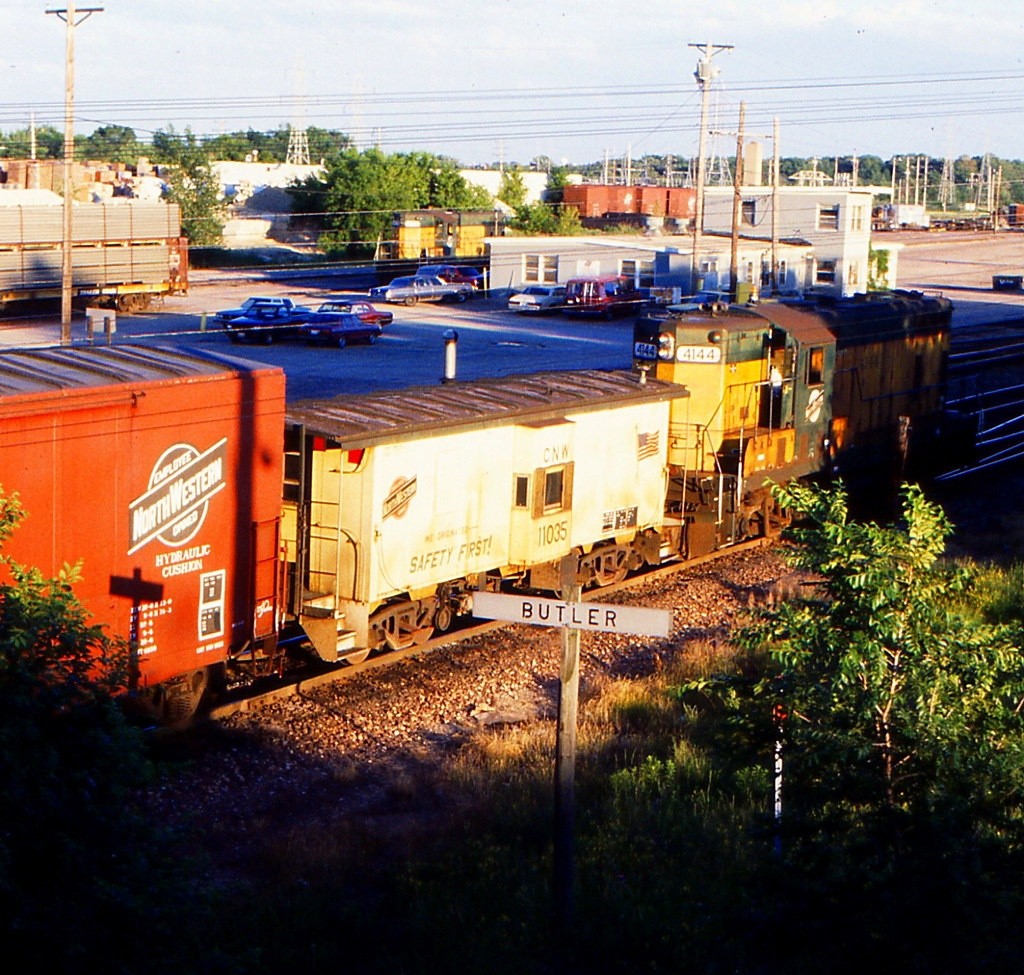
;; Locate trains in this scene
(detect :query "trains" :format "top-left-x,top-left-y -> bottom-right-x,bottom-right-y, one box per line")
0,287 -> 979,733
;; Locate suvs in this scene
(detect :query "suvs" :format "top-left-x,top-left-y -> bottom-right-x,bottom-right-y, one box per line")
562,274 -> 643,319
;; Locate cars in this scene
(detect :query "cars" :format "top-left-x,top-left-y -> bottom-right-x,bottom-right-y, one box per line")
213,295 -> 393,347
369,276 -> 470,305
508,285 -> 566,313
417,264 -> 489,289
